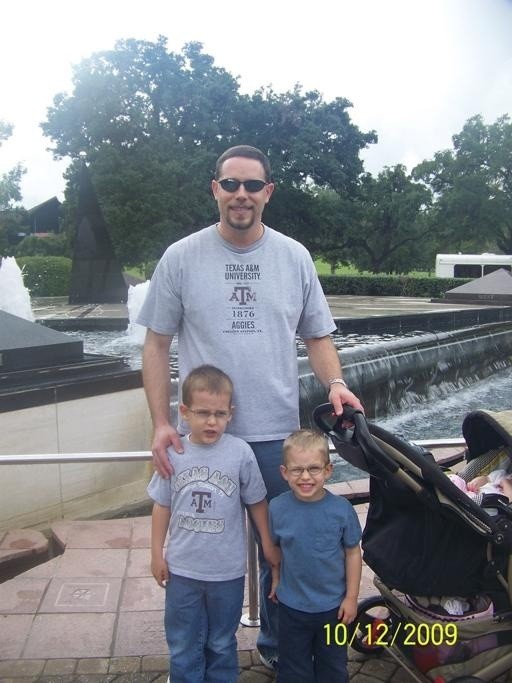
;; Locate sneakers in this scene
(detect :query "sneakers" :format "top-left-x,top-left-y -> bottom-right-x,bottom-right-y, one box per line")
260,654 -> 280,671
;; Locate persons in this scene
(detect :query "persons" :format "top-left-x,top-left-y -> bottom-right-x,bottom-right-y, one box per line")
147,364 -> 280,683
268,431 -> 362,682
137,144 -> 365,667
442,463 -> 512,506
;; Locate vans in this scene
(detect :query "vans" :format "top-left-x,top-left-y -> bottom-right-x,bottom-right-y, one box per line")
436,254 -> 512,280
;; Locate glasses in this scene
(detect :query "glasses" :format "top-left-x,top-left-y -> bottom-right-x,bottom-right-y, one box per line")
284,460 -> 330,475
189,409 -> 227,417
218,178 -> 269,192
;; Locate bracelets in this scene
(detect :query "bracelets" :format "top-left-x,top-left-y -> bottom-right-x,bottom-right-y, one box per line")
328,378 -> 346,393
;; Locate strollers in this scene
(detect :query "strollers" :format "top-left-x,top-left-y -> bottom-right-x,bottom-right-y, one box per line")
312,403 -> 512,683
